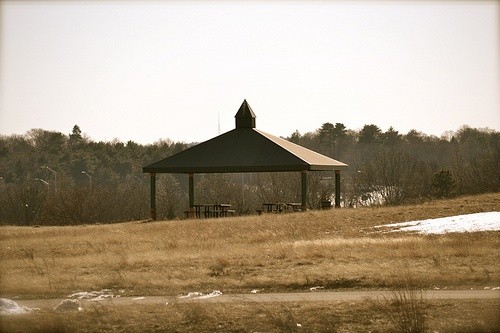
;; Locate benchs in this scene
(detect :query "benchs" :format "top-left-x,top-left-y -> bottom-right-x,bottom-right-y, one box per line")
255,209 -> 283,215
184,210 -> 235,219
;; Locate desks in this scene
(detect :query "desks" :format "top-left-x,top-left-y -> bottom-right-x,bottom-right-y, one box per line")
192,204 -> 214,221
287,203 -> 301,210
212,204 -> 232,217
262,203 -> 284,214
